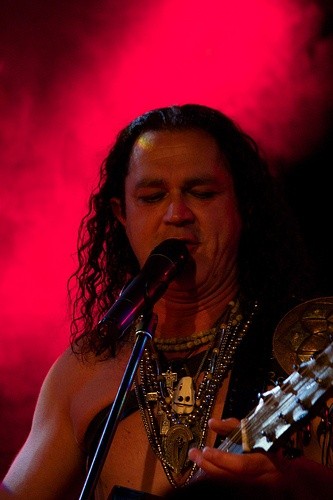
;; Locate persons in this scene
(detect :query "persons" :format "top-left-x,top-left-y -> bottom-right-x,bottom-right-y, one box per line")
0,101 -> 333,500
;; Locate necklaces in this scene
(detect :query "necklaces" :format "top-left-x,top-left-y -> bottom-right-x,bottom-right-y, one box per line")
126,290 -> 267,490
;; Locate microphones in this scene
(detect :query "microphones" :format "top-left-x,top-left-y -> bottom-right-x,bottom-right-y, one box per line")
95,237 -> 192,340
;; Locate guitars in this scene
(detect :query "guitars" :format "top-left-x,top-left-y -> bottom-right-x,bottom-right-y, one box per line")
199,341 -> 333,480
